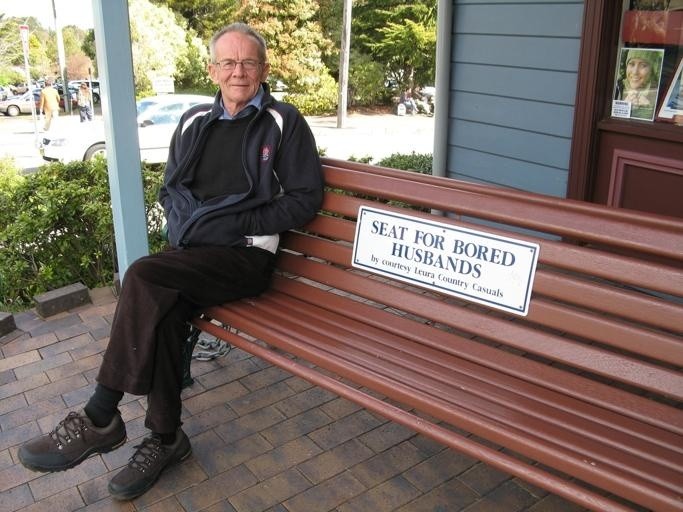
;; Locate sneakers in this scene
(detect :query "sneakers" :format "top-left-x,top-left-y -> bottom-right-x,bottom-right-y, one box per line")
18,408 -> 127,473
106,429 -> 192,502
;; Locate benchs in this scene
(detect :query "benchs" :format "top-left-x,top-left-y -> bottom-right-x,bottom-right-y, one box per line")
177,156 -> 683,512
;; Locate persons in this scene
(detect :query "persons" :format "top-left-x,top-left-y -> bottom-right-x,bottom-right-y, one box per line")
400,87 -> 419,115
37,76 -> 60,131
76,83 -> 93,121
412,85 -> 433,117
17,22 -> 325,501
616,48 -> 661,108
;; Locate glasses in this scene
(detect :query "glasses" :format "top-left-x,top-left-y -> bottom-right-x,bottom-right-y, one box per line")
214,58 -> 266,70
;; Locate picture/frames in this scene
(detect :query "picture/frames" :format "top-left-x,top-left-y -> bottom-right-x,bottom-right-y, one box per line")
655,56 -> 683,126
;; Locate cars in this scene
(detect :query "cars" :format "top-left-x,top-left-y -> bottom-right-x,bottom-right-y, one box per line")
40,94 -> 218,164
0,80 -> 100,116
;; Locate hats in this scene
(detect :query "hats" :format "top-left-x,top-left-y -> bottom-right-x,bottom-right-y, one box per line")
625,49 -> 660,82
